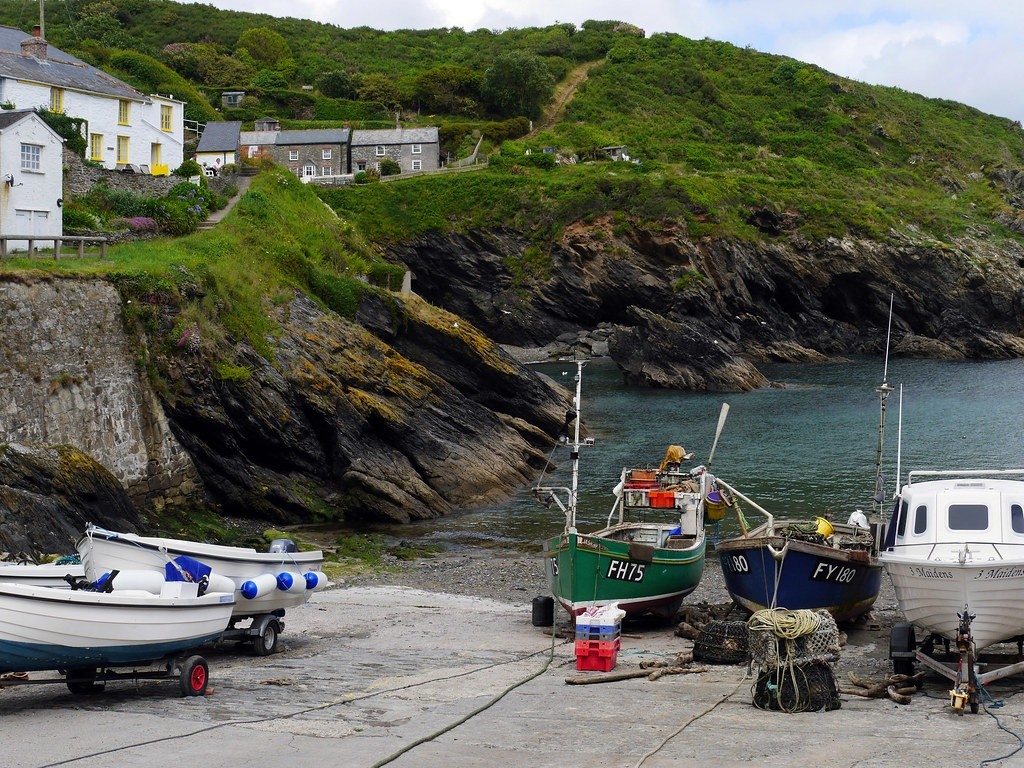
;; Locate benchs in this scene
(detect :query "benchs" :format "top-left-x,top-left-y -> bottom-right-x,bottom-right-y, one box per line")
201,165 -> 214,177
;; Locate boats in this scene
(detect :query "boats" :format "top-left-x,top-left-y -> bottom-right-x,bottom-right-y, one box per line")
74,522 -> 328,656
0,542 -> 237,698
531,359 -> 731,623
880,381 -> 1024,717
715,290 -> 895,626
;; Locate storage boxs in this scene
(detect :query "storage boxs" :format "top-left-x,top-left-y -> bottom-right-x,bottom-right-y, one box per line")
575,617 -> 621,672
160,556 -> 211,600
623,469 -> 700,509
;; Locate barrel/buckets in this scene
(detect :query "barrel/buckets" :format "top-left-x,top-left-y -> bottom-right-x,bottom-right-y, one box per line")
532,595 -> 554,627
815,516 -> 834,539
705,491 -> 727,520
